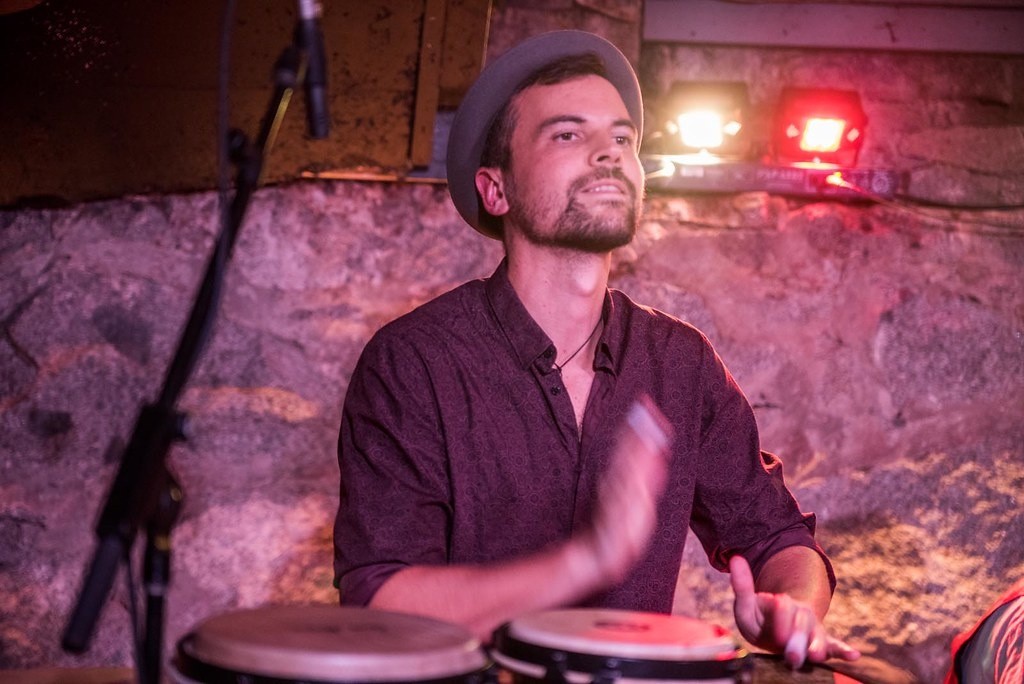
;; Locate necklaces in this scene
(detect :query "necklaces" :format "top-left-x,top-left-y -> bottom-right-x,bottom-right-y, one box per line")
554,312 -> 602,379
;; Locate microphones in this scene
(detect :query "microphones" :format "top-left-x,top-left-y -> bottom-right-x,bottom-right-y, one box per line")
300,0 -> 332,139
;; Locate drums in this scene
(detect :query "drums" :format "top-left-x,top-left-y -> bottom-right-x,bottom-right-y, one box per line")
489,609 -> 751,684
169,604 -> 491,684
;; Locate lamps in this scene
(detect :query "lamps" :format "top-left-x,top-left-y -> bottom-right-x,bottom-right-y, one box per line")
771,85 -> 868,169
661,80 -> 750,159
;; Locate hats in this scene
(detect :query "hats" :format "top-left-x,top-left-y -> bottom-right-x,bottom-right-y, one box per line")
448,30 -> 644,241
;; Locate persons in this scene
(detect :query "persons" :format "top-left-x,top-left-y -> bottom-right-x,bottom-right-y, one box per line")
334,28 -> 861,669
943,582 -> 1024,684
